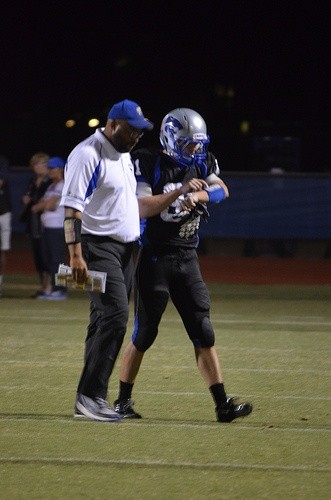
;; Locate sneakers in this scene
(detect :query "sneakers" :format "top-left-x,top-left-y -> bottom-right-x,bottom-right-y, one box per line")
73,405 -> 87,417
215,397 -> 252,422
76,393 -> 124,421
113,399 -> 142,419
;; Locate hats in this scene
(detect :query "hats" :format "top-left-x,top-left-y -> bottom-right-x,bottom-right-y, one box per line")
108,100 -> 153,129
45,156 -> 65,169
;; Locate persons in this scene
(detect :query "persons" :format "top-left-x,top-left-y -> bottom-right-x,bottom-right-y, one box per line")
32,158 -> 64,298
23,153 -> 51,298
60,99 -> 153,419
0,178 -> 11,284
115,107 -> 253,423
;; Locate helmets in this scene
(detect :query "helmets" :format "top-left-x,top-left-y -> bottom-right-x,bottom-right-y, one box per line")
159,108 -> 209,166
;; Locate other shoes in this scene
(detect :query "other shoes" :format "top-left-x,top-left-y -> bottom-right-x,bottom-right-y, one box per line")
46,290 -> 69,300
34,290 -> 50,299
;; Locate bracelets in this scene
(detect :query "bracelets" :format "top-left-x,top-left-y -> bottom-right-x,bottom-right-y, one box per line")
205,183 -> 226,203
63,217 -> 83,244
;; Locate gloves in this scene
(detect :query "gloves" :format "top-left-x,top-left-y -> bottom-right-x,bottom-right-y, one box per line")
182,203 -> 210,223
